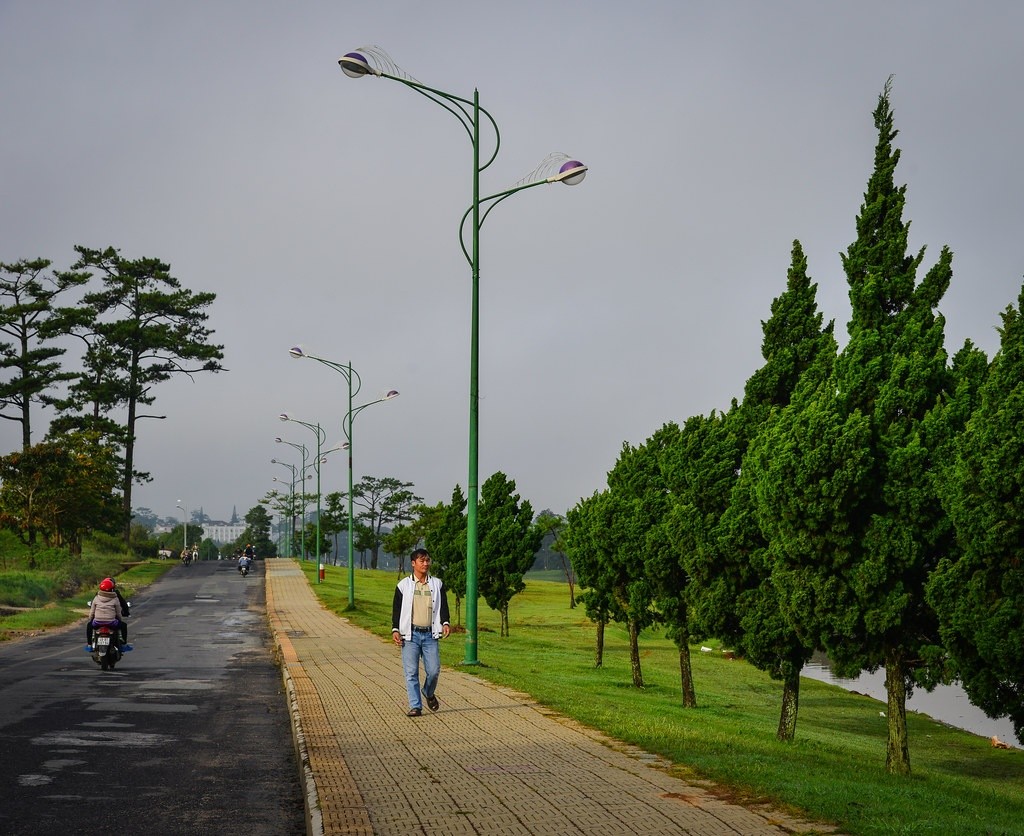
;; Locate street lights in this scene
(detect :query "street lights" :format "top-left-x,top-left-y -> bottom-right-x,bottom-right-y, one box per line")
270,458 -> 312,558
289,347 -> 400,612
336,42 -> 589,667
274,437 -> 328,562
279,413 -> 349,585
176,499 -> 187,551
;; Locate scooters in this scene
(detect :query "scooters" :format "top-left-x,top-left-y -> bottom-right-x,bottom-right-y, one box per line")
240,565 -> 249,578
183,555 -> 191,568
192,548 -> 199,562
86,601 -> 133,671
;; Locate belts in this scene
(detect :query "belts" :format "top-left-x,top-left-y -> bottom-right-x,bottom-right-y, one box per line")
411,627 -> 431,634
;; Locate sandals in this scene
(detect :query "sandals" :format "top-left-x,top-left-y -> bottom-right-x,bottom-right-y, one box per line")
421,689 -> 439,712
407,708 -> 422,716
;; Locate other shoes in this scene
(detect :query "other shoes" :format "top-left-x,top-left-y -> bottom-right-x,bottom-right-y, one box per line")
84,646 -> 92,652
122,645 -> 133,651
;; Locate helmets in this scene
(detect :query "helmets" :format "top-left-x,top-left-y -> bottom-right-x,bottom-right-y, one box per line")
100,578 -> 115,593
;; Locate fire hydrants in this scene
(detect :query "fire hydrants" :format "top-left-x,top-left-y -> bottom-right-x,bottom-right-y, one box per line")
319,564 -> 326,582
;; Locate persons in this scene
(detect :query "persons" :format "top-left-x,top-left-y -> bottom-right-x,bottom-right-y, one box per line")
391,548 -> 451,716
238,554 -> 250,572
83,574 -> 133,652
193,542 -> 199,559
182,546 -> 191,562
244,544 -> 253,556
